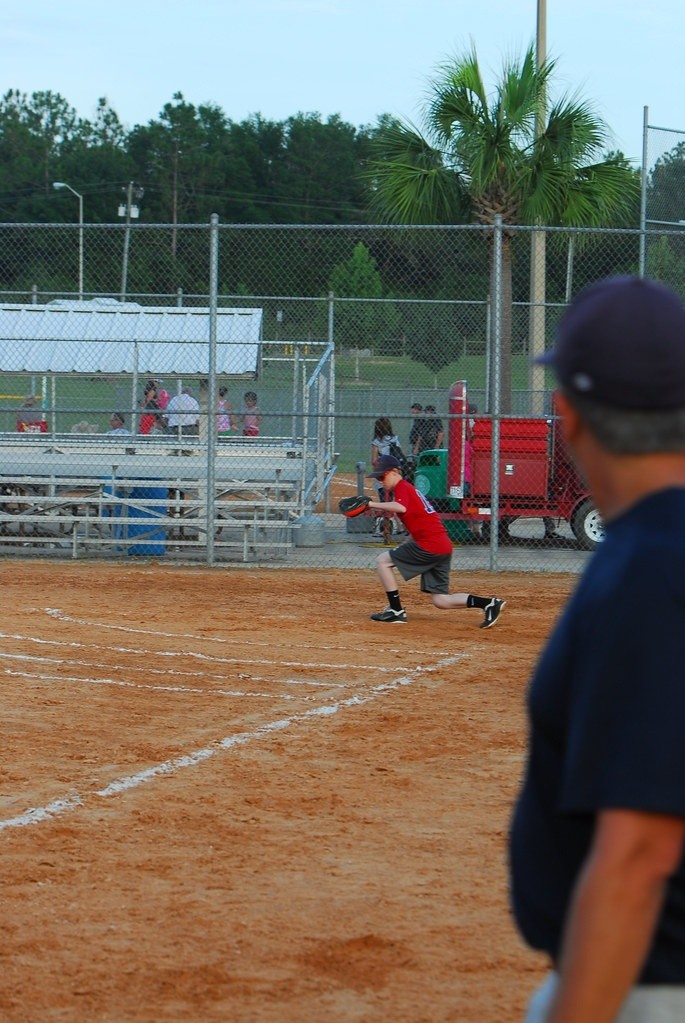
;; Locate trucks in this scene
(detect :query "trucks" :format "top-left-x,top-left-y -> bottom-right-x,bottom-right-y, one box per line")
446,380 -> 608,552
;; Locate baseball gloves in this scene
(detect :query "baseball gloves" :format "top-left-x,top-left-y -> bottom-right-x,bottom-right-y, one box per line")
338,495 -> 373,518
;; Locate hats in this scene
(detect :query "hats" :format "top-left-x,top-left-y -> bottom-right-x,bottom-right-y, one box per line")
410,403 -> 423,411
423,405 -> 436,411
533,276 -> 684,408
365,455 -> 400,478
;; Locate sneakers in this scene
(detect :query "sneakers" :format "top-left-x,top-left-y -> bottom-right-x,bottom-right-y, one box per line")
479,597 -> 506,628
369,608 -> 408,623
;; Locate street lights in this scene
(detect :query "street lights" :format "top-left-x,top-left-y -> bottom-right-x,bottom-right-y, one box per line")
53,182 -> 82,300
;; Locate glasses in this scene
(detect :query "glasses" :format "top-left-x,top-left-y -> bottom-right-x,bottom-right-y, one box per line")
376,470 -> 392,481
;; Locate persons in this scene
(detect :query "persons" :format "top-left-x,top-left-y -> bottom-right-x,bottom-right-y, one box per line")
163,386 -> 199,435
140,378 -> 168,433
507,276 -> 685,1023
218,385 -> 239,436
230,392 -> 262,436
468,404 -> 478,437
339,455 -> 506,629
371,418 -> 409,536
409,403 -> 444,466
106,413 -> 130,434
498,514 -> 566,540
16,395 -> 49,433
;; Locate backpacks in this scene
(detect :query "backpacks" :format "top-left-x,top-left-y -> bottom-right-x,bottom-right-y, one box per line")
377,439 -> 409,481
